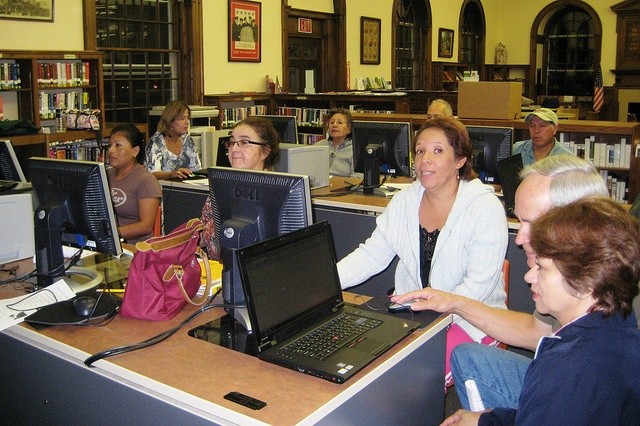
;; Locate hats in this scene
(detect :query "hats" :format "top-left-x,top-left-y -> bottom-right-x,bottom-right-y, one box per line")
524,108 -> 558,125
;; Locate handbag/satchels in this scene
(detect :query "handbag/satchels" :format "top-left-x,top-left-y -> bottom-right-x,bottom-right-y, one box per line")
0,119 -> 41,136
67,107 -> 102,130
116,218 -> 211,322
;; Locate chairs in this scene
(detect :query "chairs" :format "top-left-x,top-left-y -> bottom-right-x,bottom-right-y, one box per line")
153,207 -> 162,237
441,260 -> 511,393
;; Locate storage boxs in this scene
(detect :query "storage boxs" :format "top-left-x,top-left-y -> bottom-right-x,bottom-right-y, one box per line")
455,80 -> 526,121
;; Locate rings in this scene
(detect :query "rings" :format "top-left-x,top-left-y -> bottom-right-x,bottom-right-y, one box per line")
177,171 -> 181,175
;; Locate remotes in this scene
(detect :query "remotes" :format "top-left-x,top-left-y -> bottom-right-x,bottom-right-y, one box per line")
223,390 -> 267,411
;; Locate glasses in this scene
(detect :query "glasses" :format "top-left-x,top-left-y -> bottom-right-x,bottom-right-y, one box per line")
224,139 -> 266,148
328,119 -> 349,125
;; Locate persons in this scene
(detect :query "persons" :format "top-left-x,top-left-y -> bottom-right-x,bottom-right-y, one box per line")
232,16 -> 258,43
335,116 -> 508,394
512,107 -> 573,168
427,99 -> 453,122
440,197 -> 640,426
313,108 -> 365,180
106,123 -> 163,246
143,100 -> 202,180
390,154 -> 612,412
198,116 -> 281,261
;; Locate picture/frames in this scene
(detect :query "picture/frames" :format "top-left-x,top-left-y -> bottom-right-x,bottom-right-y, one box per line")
437,27 -> 455,60
227,0 -> 262,64
360,15 -> 382,66
0,0 -> 57,24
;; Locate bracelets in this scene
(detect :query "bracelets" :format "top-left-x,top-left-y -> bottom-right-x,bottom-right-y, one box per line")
169,171 -> 174,180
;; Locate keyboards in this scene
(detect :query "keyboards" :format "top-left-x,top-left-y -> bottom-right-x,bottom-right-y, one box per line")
197,168 -> 208,176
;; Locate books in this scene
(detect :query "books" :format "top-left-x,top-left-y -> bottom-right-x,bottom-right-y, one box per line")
298,133 -> 324,146
223,105 -> 268,128
0,62 -> 22,90
599,170 -> 629,203
353,109 -> 395,114
558,132 -> 632,170
49,138 -> 110,167
277,106 -> 329,127
37,62 -> 90,89
196,257 -> 226,297
38,92 -> 90,121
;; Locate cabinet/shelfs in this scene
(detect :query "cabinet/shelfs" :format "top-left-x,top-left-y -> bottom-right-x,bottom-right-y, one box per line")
413,60 -> 538,117
203,91 -> 412,130
0,49 -> 151,159
352,112 -> 639,203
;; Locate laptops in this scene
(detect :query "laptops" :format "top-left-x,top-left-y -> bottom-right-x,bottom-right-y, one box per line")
496,153 -> 540,219
236,220 -> 422,383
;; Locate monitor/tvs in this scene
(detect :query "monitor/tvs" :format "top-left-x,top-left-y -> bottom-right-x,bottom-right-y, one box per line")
27,157 -> 123,295
216,135 -> 230,167
247,114 -> 298,145
0,140 -> 27,184
207,165 -> 313,331
351,120 -> 412,198
465,125 -> 513,196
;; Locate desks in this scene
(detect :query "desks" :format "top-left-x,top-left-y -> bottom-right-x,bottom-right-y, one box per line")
157,172 -> 535,315
0,243 -> 445,426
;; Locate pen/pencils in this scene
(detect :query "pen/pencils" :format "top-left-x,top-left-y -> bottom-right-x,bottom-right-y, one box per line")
95,289 -> 125,293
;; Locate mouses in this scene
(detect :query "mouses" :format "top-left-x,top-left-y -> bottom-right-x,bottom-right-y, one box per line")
386,300 -> 420,313
71,295 -> 95,317
185,171 -> 195,177
